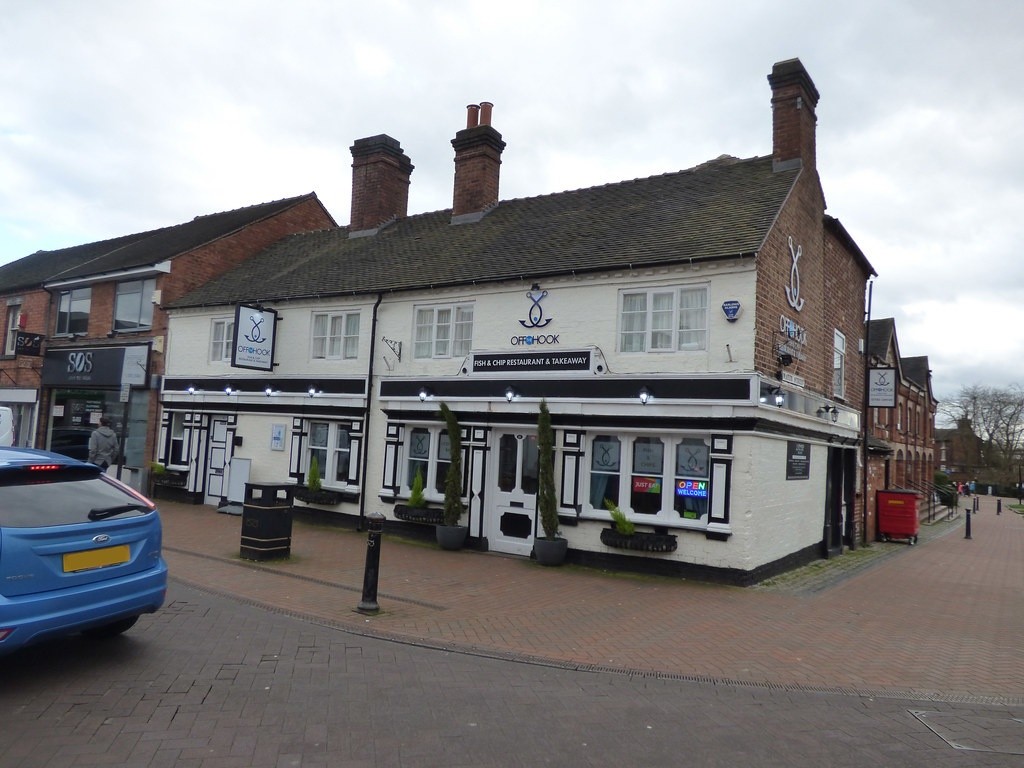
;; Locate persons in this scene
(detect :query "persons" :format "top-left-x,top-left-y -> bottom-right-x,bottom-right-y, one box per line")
952,480 -> 976,496
88,418 -> 120,472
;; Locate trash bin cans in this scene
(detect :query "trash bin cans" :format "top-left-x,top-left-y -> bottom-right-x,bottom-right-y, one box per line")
876,489 -> 923,545
987,484 -> 998,495
239,482 -> 296,562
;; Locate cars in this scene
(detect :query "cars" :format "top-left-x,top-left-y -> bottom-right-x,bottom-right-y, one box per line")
0,448 -> 164,666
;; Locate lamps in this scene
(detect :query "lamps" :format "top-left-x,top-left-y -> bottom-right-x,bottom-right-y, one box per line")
816,407 -> 826,420
188,382 -> 196,395
638,386 -> 649,406
308,384 -> 317,399
768,384 -> 785,407
265,383 -> 273,398
418,386 -> 428,402
826,405 -> 839,422
225,384 -> 232,396
504,384 -> 514,402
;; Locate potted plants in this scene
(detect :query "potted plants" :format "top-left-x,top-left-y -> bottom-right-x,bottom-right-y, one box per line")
435,403 -> 469,550
394,467 -> 443,523
293,457 -> 341,505
150,463 -> 188,487
534,396 -> 567,567
